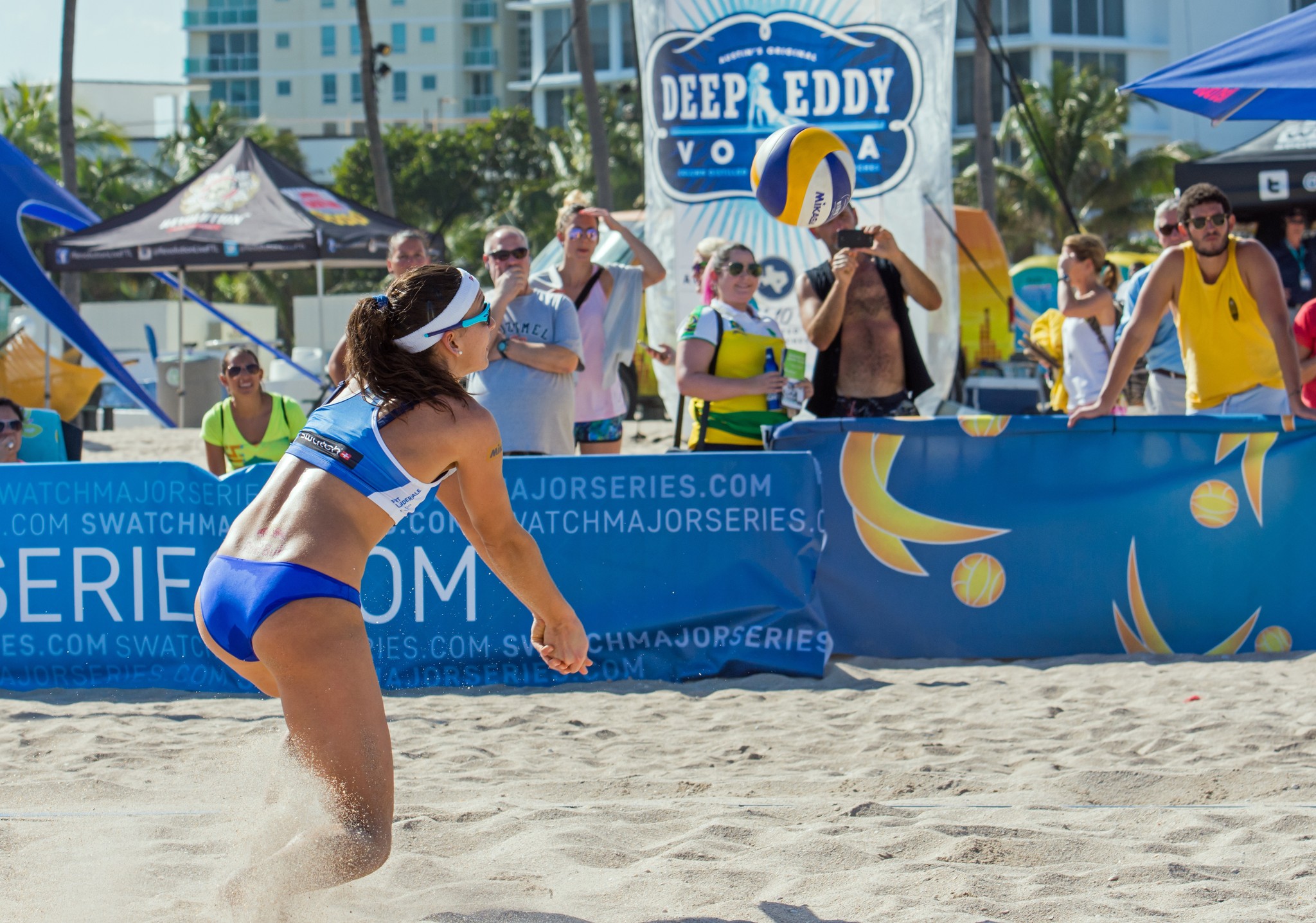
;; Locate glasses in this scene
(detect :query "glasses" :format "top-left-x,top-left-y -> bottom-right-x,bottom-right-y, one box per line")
1184,212 -> 1228,230
1156,222 -> 1183,236
424,303 -> 490,337
562,226 -> 600,243
721,262 -> 762,278
0,420 -> 23,433
226,363 -> 259,378
487,247 -> 528,262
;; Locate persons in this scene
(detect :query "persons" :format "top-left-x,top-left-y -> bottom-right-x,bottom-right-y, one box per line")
328,228 -> 431,387
1055,181 -> 1316,428
0,397 -> 27,462
201,346 -> 308,475
461,225 -> 588,456
797,202 -> 944,418
191,260 -> 592,923
528,203 -> 668,455
672,236 -> 813,451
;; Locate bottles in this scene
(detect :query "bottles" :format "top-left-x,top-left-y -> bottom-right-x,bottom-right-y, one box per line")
763,346 -> 782,411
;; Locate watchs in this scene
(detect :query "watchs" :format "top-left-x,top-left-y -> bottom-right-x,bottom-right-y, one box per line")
497,336 -> 510,359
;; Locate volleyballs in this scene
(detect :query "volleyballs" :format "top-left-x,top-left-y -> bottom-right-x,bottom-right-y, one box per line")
748,123 -> 856,229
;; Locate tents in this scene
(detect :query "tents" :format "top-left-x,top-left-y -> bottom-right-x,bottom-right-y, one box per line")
1117,0 -> 1316,128
40,134 -> 450,409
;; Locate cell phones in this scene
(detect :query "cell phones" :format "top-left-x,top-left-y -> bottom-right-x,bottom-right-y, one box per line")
836,229 -> 874,248
638,340 -> 668,354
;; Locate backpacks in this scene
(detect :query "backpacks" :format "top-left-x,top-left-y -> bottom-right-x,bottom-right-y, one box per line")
1087,296 -> 1150,406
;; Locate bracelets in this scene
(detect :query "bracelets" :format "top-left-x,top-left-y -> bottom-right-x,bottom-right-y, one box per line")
1057,274 -> 1070,281
1288,389 -> 1302,397
